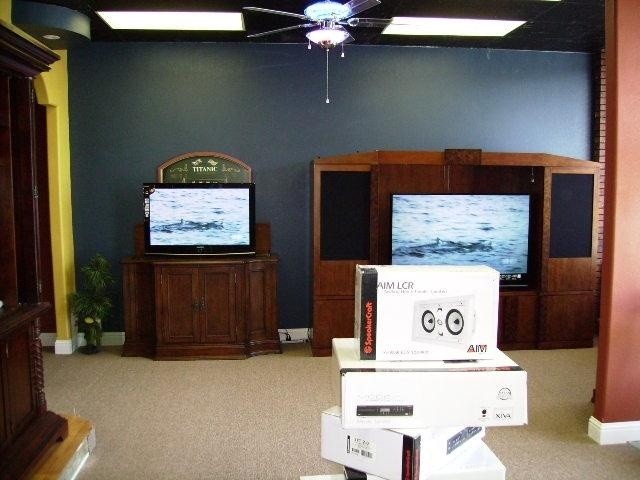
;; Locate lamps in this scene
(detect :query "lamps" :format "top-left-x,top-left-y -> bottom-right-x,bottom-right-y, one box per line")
300,21 -> 354,106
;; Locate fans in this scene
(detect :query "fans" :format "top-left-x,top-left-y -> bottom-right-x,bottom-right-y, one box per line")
241,0 -> 400,47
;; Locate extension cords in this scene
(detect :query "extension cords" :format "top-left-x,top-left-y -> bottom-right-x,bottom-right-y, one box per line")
281,339 -> 304,344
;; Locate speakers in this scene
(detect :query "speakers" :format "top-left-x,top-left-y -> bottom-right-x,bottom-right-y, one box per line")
320,171 -> 371,260
549,174 -> 594,258
412,297 -> 477,346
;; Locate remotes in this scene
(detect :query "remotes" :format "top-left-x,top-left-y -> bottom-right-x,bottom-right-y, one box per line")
357,405 -> 413,416
447,427 -> 482,455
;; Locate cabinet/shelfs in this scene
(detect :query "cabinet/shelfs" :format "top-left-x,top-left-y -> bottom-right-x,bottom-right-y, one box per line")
311,148 -> 598,363
0,20 -> 69,479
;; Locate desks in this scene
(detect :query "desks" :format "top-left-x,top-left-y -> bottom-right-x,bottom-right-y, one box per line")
120,255 -> 286,365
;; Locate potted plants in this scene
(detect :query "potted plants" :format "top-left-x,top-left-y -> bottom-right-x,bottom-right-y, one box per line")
64,251 -> 112,358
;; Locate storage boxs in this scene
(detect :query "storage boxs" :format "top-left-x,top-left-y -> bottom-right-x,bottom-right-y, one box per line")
319,404 -> 485,480
354,263 -> 499,361
343,438 -> 506,479
331,338 -> 527,430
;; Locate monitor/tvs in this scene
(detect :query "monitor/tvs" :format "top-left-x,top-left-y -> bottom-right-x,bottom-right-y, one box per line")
389,192 -> 531,288
143,182 -> 255,258
